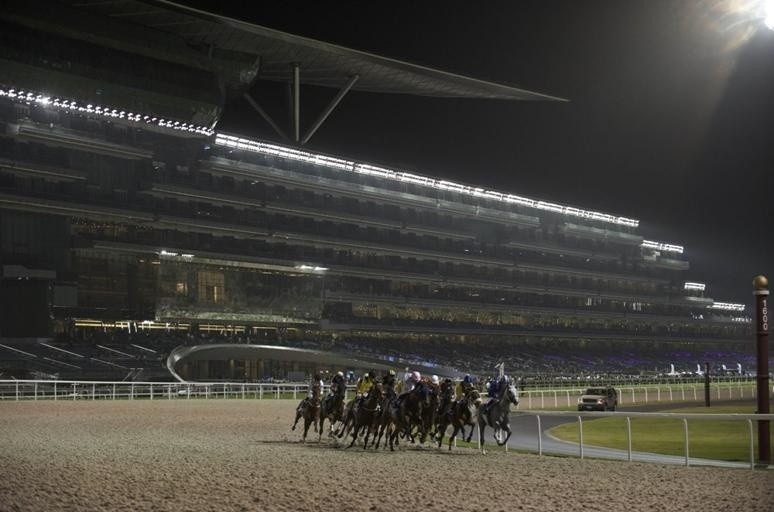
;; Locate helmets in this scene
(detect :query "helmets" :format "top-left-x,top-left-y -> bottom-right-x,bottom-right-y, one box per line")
314,370 -> 510,387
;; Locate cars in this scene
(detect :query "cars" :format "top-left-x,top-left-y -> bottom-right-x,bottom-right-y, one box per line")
577,386 -> 618,412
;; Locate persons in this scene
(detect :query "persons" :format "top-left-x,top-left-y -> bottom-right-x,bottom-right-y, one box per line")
297,370 -> 510,415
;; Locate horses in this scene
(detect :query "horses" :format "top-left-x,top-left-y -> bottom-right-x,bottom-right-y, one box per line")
292,379 -> 519,455
